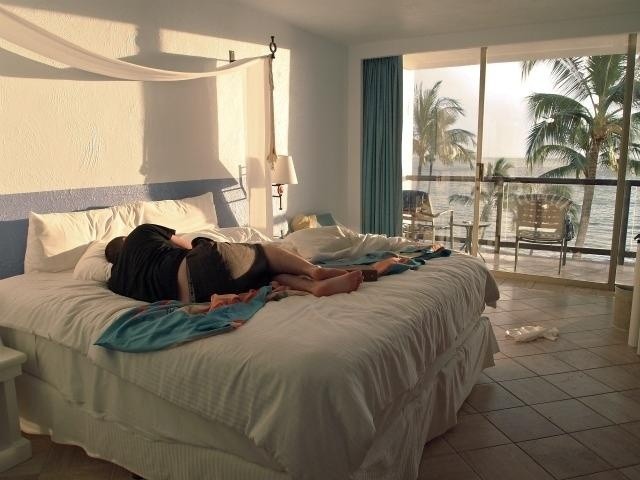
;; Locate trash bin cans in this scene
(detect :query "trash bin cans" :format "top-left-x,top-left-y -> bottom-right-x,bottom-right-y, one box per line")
612,285 -> 633,332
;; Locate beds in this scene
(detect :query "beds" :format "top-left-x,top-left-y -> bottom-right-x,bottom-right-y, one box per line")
0,222 -> 500,479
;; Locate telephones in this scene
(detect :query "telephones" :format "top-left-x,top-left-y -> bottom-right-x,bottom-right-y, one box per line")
279,230 -> 285,239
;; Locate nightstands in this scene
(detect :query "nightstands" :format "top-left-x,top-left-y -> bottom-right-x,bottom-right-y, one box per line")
0,342 -> 36,473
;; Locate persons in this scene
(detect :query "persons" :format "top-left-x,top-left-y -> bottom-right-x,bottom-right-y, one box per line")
105,224 -> 365,304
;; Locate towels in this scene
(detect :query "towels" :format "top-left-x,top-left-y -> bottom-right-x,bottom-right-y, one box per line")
505,323 -> 561,343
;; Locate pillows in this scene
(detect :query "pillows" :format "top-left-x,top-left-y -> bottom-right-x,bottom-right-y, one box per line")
21,204 -> 133,277
73,210 -> 136,289
132,191 -> 221,234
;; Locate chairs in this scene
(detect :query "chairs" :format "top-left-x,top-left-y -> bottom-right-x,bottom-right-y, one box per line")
401,187 -> 457,253
511,193 -> 572,274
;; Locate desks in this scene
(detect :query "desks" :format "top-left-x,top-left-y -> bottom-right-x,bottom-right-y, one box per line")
451,218 -> 491,265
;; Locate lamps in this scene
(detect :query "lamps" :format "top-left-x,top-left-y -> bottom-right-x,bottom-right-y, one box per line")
271,149 -> 300,213
